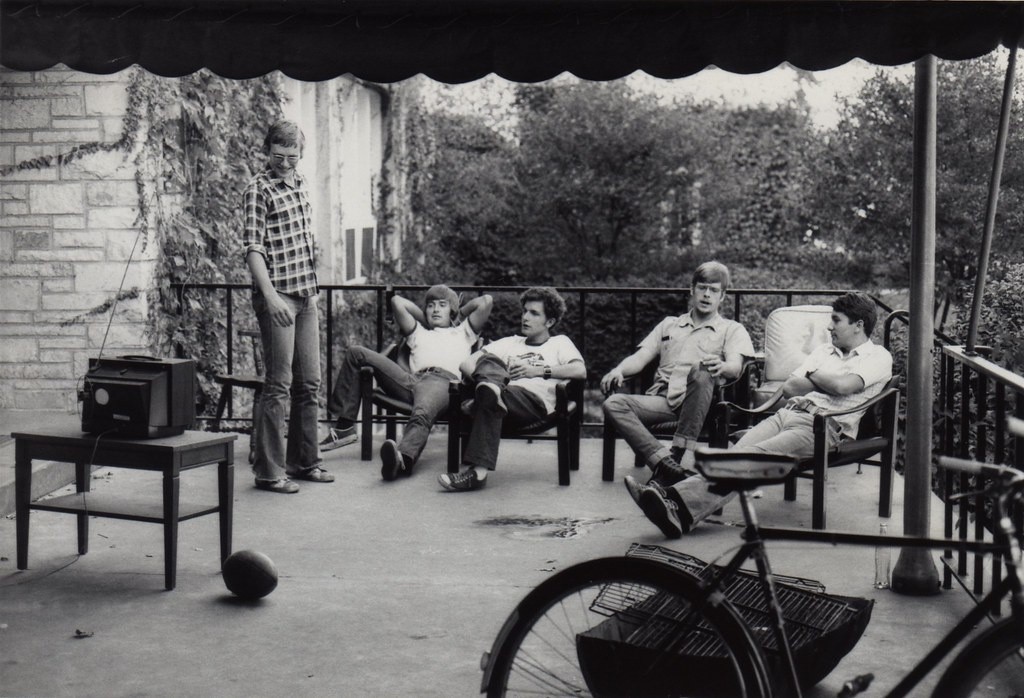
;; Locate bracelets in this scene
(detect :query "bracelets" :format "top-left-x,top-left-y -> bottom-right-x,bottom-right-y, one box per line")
805,368 -> 818,377
543,366 -> 551,379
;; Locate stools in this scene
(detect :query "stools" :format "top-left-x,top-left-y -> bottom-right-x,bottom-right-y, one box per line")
212,374 -> 265,464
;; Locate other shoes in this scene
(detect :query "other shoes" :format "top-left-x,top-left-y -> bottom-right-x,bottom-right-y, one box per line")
645,455 -> 697,487
255,478 -> 300,493
646,469 -> 723,516
285,467 -> 335,482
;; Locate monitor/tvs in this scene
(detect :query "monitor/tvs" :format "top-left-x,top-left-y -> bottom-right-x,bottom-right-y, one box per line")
82,189 -> 197,439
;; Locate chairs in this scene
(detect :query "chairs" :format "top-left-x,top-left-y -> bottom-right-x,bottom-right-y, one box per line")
602,304 -> 901,530
359,321 -> 583,486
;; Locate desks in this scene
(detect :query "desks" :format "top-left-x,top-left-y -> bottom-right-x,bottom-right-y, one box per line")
11,422 -> 238,589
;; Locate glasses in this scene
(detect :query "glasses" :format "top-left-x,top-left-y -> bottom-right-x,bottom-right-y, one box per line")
270,151 -> 300,164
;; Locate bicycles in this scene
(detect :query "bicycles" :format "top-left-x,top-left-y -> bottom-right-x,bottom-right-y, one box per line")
475,442 -> 1024,698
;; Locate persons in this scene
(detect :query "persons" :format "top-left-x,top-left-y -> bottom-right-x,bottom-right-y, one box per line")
601,263 -> 754,481
625,296 -> 894,540
243,121 -> 335,492
320,284 -> 494,481
438,286 -> 587,492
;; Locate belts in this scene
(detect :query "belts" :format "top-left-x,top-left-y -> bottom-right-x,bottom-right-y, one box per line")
787,402 -> 845,441
419,367 -> 458,381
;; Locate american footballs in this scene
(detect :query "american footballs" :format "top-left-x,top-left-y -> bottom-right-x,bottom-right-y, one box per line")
222,550 -> 279,600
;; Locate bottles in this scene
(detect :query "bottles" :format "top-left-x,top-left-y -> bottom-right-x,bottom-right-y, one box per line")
874,523 -> 891,589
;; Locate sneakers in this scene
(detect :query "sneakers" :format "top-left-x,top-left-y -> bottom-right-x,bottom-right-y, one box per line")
624,475 -> 682,539
475,382 -> 507,418
319,426 -> 358,450
380,439 -> 405,481
438,465 -> 487,491
